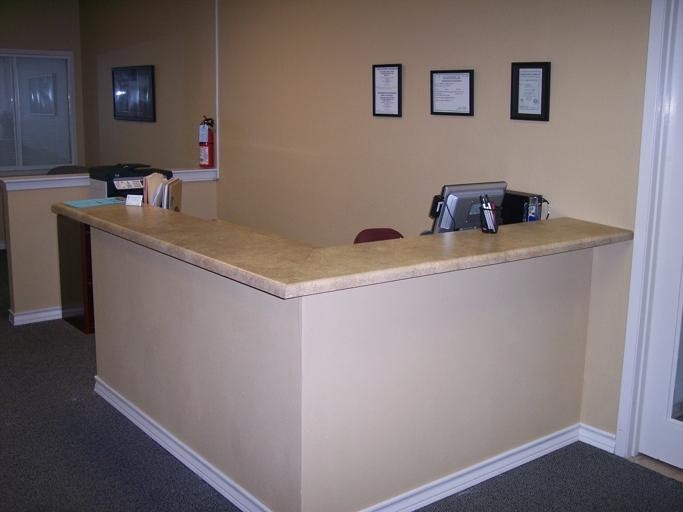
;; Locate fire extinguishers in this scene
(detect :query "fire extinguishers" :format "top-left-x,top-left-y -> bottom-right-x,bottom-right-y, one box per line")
199,115 -> 214,168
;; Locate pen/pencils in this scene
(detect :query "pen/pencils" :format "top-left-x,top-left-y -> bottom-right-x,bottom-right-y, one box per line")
479,194 -> 498,232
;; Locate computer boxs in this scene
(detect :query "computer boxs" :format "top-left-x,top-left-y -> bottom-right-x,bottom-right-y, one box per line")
501,190 -> 543,225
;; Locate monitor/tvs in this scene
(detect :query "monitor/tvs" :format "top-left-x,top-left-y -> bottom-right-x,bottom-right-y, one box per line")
432,181 -> 508,233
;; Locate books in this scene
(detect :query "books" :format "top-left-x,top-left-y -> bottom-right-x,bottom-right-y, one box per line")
141,171 -> 182,213
